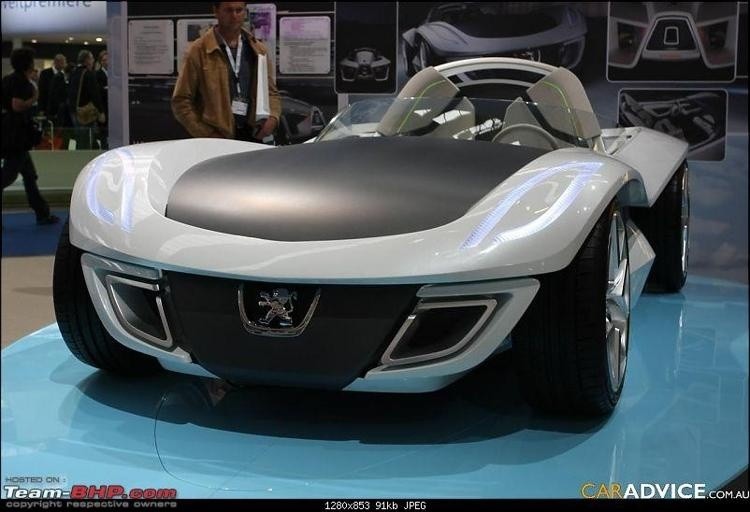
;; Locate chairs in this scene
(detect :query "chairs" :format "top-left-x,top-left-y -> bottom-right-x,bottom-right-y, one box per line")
377,64 -> 601,152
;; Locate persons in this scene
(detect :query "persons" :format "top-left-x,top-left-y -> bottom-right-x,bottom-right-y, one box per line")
28,49 -> 109,151
169,0 -> 283,146
0,47 -> 62,228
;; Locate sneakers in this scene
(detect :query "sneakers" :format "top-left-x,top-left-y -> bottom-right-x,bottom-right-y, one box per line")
35,215 -> 63,225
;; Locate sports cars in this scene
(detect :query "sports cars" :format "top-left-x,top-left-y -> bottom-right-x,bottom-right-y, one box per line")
52,57 -> 692,418
401,0 -> 593,80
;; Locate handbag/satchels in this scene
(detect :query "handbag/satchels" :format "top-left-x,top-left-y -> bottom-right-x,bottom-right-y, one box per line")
76,103 -> 99,127
